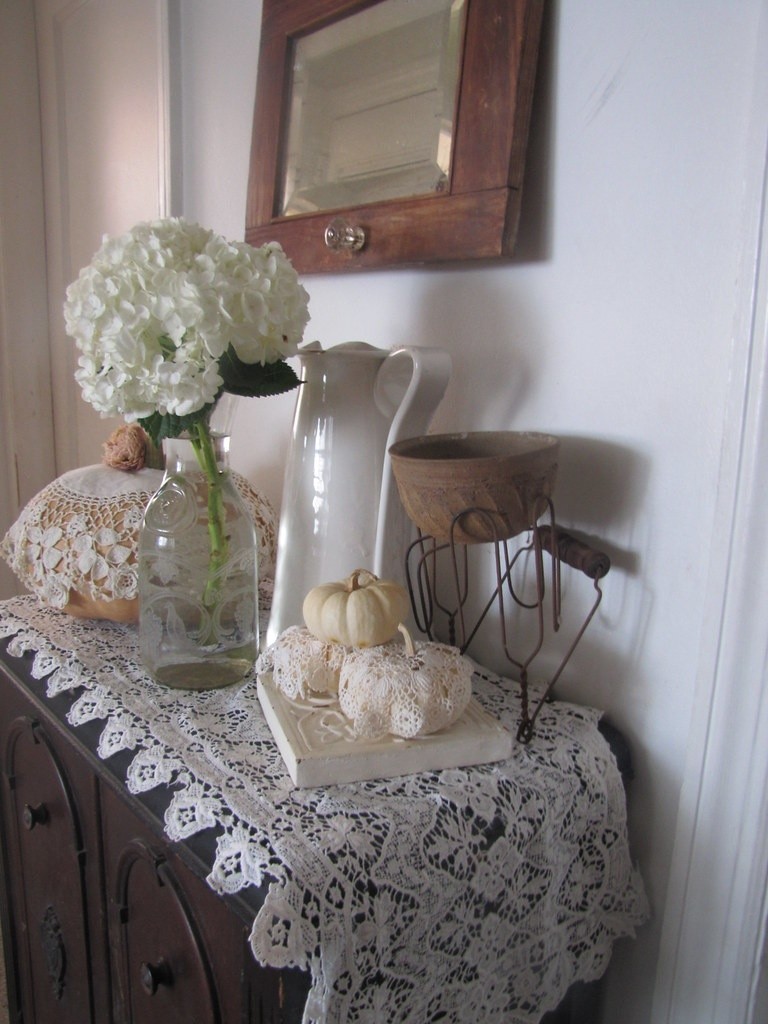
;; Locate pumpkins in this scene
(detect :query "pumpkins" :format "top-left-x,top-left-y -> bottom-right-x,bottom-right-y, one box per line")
341,624 -> 464,737
304,570 -> 409,647
273,624 -> 346,697
27,472 -> 270,626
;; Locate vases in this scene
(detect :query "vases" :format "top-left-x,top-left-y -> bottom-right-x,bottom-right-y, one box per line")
140,435 -> 259,691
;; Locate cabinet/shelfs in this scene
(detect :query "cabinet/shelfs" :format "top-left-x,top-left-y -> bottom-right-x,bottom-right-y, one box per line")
0,596 -> 652,1024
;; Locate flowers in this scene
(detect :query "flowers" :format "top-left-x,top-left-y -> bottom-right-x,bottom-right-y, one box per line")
65,216 -> 311,642
